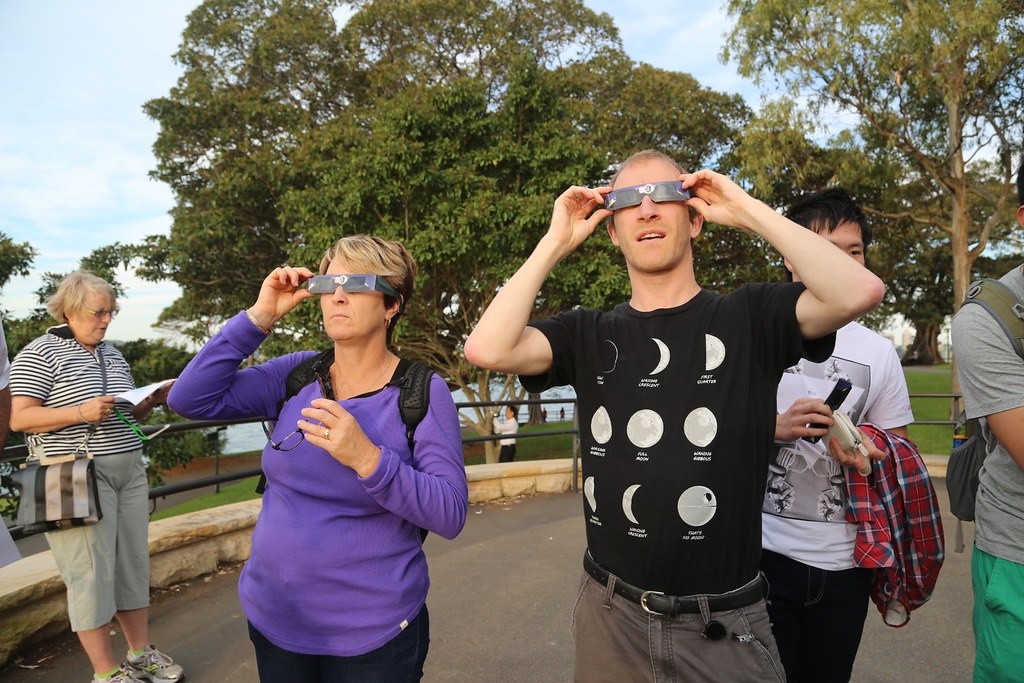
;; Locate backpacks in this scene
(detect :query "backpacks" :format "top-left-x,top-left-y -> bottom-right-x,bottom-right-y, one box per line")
945,277 -> 1024,521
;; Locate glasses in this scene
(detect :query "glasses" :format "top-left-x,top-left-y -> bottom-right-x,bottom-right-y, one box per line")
78,306 -> 120,320
801,379 -> 852,444
604,181 -> 691,210
300,273 -> 403,314
261,372 -> 330,452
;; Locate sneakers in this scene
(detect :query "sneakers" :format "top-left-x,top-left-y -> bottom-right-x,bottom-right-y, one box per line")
93,668 -> 145,683
126,645 -> 185,683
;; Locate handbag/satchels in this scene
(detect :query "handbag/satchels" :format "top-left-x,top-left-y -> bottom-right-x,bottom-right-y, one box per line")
12,458 -> 104,534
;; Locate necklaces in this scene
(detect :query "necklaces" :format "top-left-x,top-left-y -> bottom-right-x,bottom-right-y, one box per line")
333,352 -> 393,399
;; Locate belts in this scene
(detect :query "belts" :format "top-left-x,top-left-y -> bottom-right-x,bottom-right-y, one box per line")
584,548 -> 764,618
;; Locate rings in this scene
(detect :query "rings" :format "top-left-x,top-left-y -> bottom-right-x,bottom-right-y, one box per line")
324,427 -> 329,439
106,408 -> 111,415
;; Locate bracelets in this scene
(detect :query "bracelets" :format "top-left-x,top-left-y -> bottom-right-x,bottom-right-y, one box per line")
77,403 -> 94,425
246,309 -> 273,336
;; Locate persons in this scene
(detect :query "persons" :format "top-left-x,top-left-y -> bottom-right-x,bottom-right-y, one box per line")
493,406 -> 519,462
950,158 -> 1024,683
0,312 -> 12,455
9,270 -> 182,683
166,233 -> 469,683
559,407 -> 565,422
760,186 -> 914,683
464,150 -> 886,683
542,408 -> 547,423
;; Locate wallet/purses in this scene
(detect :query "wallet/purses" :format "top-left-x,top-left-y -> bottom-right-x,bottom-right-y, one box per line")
822,411 -> 863,455
